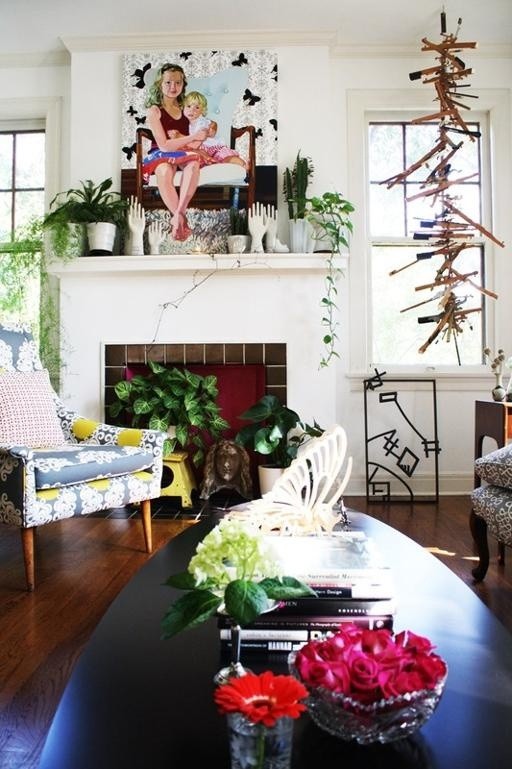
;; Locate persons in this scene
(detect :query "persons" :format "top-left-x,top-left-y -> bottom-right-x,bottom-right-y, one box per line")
169,92 -> 246,172
145,64 -> 215,243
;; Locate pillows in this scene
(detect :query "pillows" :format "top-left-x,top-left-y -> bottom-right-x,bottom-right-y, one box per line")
0,369 -> 66,450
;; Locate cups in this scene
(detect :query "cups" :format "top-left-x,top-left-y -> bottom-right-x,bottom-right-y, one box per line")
227,235 -> 248,254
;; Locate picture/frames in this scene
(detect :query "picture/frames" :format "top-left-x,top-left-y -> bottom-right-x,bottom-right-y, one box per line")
118,48 -> 281,255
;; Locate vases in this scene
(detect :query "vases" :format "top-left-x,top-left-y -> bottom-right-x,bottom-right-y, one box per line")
290,631 -> 445,747
505,377 -> 512,402
492,372 -> 505,400
211,623 -> 250,686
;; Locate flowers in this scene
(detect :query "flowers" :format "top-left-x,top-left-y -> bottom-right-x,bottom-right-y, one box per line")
157,518 -> 319,637
485,348 -> 505,373
297,624 -> 446,727
506,355 -> 512,369
41,499 -> 511,769
214,672 -> 310,767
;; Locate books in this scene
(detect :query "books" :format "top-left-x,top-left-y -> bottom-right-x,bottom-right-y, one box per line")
215,572 -> 394,664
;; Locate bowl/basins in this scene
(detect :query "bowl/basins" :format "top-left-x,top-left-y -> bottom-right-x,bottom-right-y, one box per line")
288,645 -> 450,742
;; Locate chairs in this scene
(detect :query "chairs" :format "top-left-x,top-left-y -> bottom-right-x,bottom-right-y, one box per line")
0,324 -> 167,591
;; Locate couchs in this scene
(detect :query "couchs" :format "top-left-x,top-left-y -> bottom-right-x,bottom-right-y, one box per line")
470,445 -> 511,580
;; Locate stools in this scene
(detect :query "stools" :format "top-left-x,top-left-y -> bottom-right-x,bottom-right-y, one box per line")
133,453 -> 201,510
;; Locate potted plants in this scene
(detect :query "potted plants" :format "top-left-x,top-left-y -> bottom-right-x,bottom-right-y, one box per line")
286,149 -> 308,252
305,191 -> 355,370
283,156 -> 316,253
108,359 -> 232,468
40,177 -> 135,256
1,196 -> 88,394
233,395 -> 326,498
